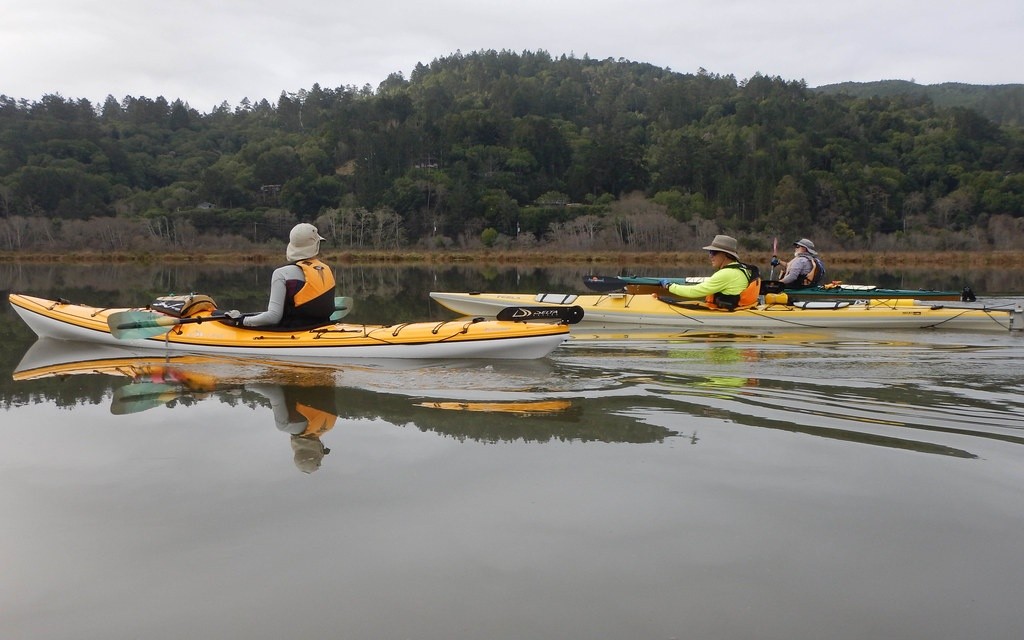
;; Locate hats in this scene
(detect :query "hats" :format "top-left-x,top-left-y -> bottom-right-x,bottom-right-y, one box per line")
703,235 -> 741,259
792,239 -> 817,255
286,223 -> 326,262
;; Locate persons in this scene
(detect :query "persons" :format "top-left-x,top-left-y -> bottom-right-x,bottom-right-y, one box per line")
657,234 -> 752,310
770,238 -> 824,288
225,223 -> 336,328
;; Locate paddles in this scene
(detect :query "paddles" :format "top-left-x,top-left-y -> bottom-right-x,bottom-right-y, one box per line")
105,294 -> 356,342
582,274 -> 787,294
768,237 -> 779,281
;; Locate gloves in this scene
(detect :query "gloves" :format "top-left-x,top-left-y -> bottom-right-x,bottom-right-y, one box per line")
660,280 -> 670,288
771,258 -> 780,267
225,310 -> 243,321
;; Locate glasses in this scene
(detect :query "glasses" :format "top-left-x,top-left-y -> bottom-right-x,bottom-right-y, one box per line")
709,250 -> 720,256
797,245 -> 803,248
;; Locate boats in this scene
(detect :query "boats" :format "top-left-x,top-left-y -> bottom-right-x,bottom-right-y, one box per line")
429,290 -> 1015,332
579,274 -> 977,305
8,287 -> 573,362
10,336 -> 649,414
